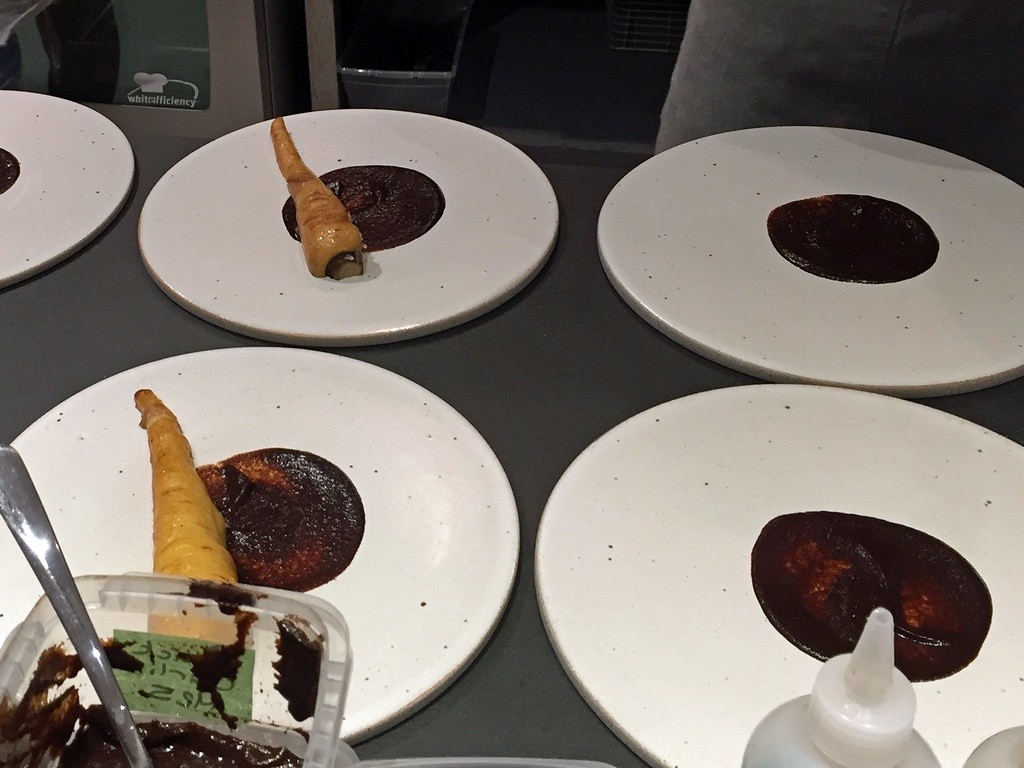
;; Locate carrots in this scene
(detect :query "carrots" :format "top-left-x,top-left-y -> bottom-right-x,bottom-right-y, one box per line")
133,388 -> 251,648
271,116 -> 365,280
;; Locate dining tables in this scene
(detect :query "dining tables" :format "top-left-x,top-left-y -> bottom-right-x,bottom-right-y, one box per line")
0,104 -> 1024,768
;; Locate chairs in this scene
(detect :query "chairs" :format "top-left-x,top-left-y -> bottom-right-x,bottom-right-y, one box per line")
656,0 -> 1024,187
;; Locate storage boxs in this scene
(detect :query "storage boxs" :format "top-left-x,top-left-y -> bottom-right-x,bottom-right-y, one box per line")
0,569 -> 358,768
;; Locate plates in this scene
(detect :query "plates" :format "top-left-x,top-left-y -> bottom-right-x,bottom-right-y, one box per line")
0,89 -> 136,290
533,383 -> 1024,768
0,347 -> 521,745
137,107 -> 560,349
597,126 -> 1024,399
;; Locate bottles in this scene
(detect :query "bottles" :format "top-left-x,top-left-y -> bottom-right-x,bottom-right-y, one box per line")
963,726 -> 1024,768
741,608 -> 942,768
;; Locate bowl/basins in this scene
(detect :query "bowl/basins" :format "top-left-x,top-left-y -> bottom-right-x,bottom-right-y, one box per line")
0,573 -> 353,768
